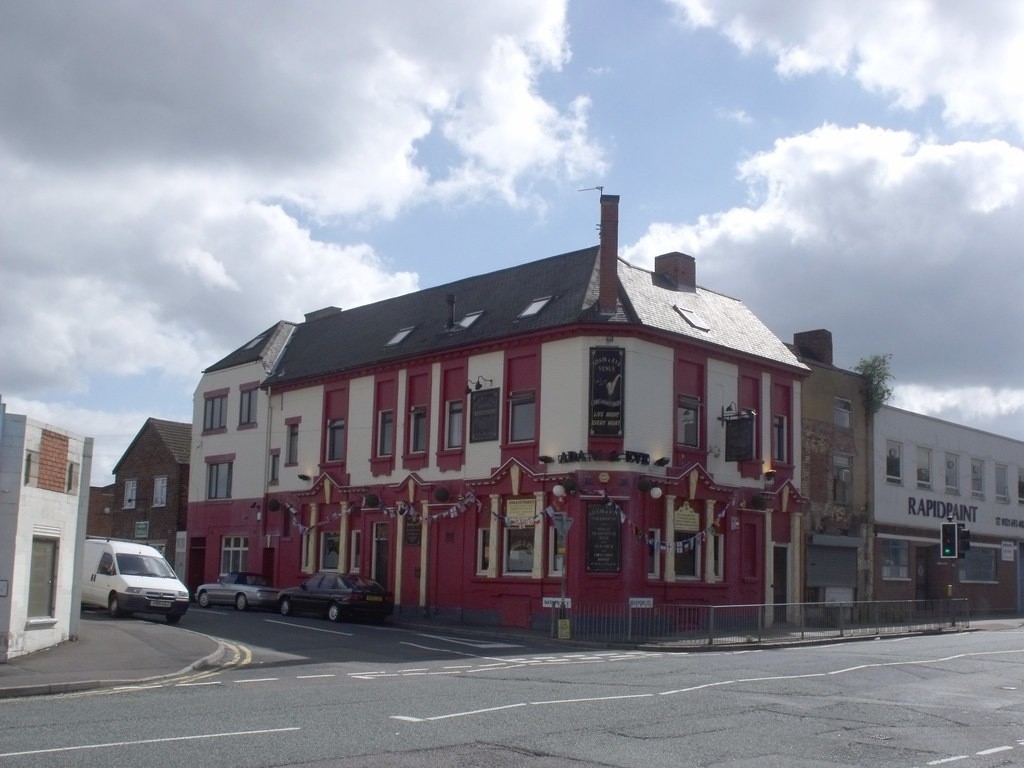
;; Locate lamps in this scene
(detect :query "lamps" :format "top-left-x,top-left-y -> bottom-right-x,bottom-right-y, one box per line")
654,456 -> 669,467
298,474 -> 310,481
465,379 -> 476,394
726,401 -> 738,414
552,480 -> 576,497
638,481 -> 662,498
475,376 -> 492,390
539,455 -> 553,465
764,469 -> 777,492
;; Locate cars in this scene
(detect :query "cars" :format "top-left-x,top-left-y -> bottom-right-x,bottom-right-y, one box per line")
194,570 -> 281,612
277,571 -> 395,624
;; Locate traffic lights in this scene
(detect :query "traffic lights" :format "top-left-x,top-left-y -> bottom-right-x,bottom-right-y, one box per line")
940,522 -> 958,559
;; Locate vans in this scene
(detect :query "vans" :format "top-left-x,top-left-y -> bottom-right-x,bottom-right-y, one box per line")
82,539 -> 190,624
